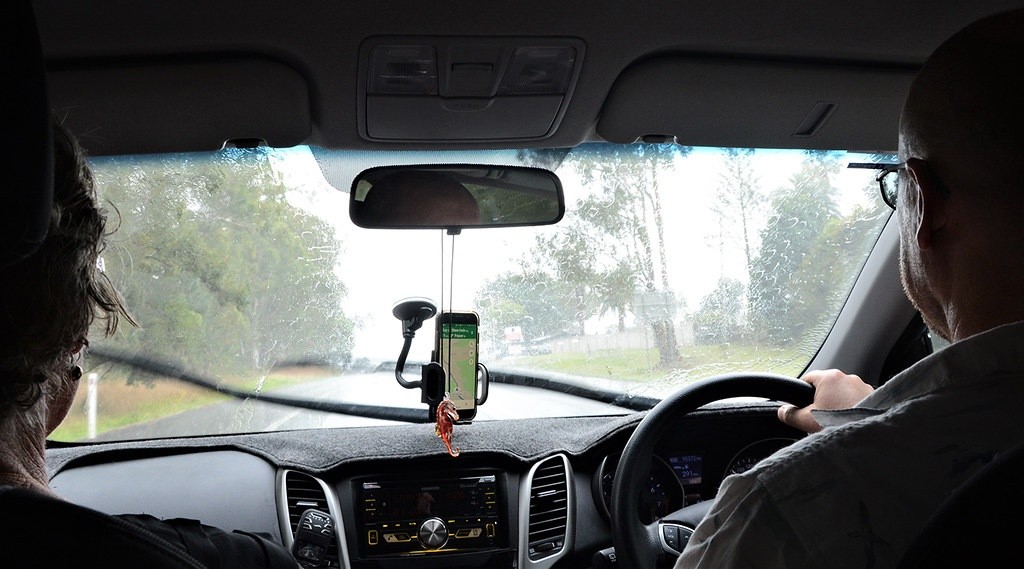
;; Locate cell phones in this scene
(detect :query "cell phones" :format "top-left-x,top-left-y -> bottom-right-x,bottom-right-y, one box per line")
435,311 -> 479,420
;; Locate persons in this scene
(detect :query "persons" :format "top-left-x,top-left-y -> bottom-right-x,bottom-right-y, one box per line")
0,88 -> 297,569
355,171 -> 482,225
675,9 -> 1024,569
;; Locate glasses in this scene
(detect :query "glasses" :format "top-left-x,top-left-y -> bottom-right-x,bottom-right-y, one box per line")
875,160 -> 950,209
